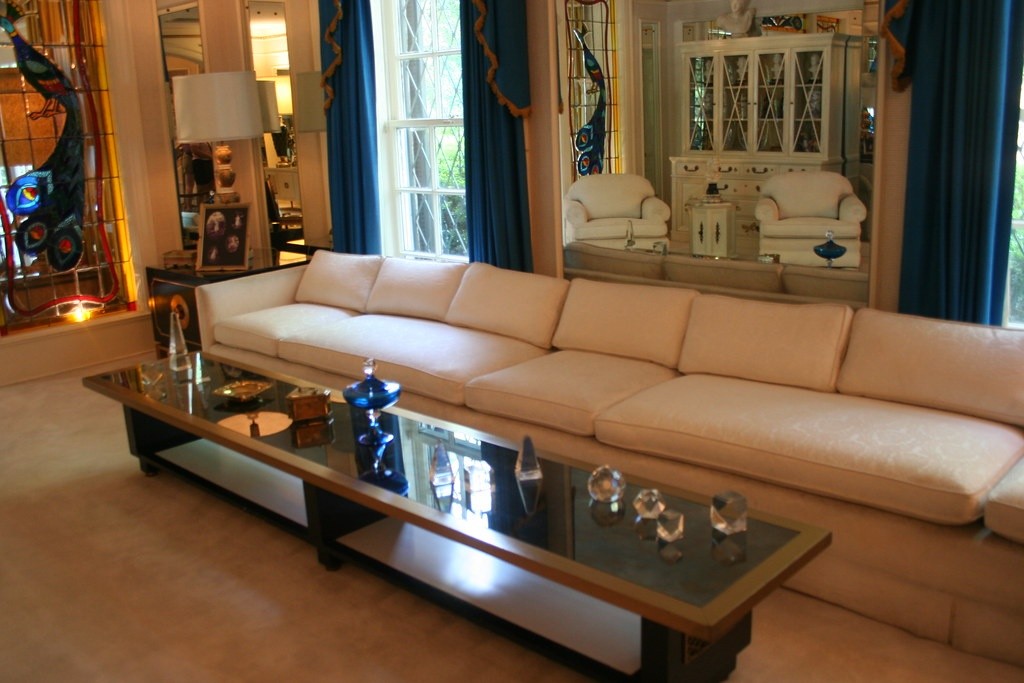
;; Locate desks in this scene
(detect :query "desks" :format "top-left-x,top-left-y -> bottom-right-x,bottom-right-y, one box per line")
146,249 -> 309,362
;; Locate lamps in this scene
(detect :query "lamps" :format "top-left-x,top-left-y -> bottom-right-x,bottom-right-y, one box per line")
171,71 -> 265,201
256,78 -> 282,134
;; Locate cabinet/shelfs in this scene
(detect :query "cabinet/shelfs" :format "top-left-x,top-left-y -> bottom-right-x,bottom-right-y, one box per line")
668,34 -> 863,263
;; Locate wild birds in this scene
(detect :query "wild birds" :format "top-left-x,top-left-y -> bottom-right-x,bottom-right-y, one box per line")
572,21 -> 606,177
0,0 -> 85,272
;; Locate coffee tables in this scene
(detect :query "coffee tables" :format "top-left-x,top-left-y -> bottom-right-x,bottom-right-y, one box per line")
82,350 -> 834,683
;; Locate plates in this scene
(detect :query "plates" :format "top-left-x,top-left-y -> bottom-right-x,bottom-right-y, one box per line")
212,381 -> 274,407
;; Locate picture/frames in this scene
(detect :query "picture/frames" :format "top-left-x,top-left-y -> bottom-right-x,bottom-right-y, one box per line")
195,202 -> 252,271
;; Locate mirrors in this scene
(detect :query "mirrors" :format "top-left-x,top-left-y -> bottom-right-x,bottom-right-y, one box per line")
240,2 -> 308,262
556,1 -> 874,312
158,7 -> 216,252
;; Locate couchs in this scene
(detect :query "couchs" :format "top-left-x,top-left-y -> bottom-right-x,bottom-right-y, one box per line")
754,172 -> 867,270
194,265 -> 1024,665
562,173 -> 672,257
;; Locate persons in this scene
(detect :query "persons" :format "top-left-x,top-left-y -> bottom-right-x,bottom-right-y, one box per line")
179,144 -> 195,211
191,142 -> 215,203
716,0 -> 756,33
